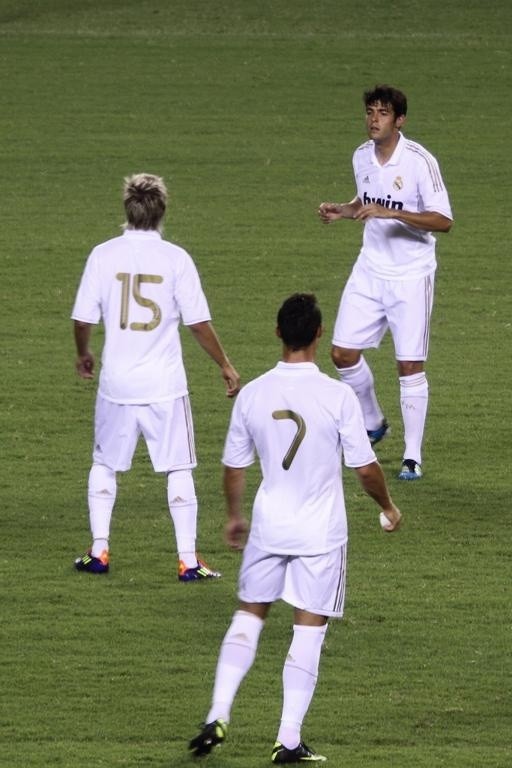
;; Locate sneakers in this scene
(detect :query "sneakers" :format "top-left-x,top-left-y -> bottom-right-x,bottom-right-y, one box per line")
365,417 -> 391,446
399,456 -> 423,480
188,719 -> 228,757
178,557 -> 221,580
272,739 -> 328,763
74,548 -> 110,573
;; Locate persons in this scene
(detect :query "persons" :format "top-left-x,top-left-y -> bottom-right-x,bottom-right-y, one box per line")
183,291 -> 405,765
314,81 -> 456,480
66,171 -> 243,579
